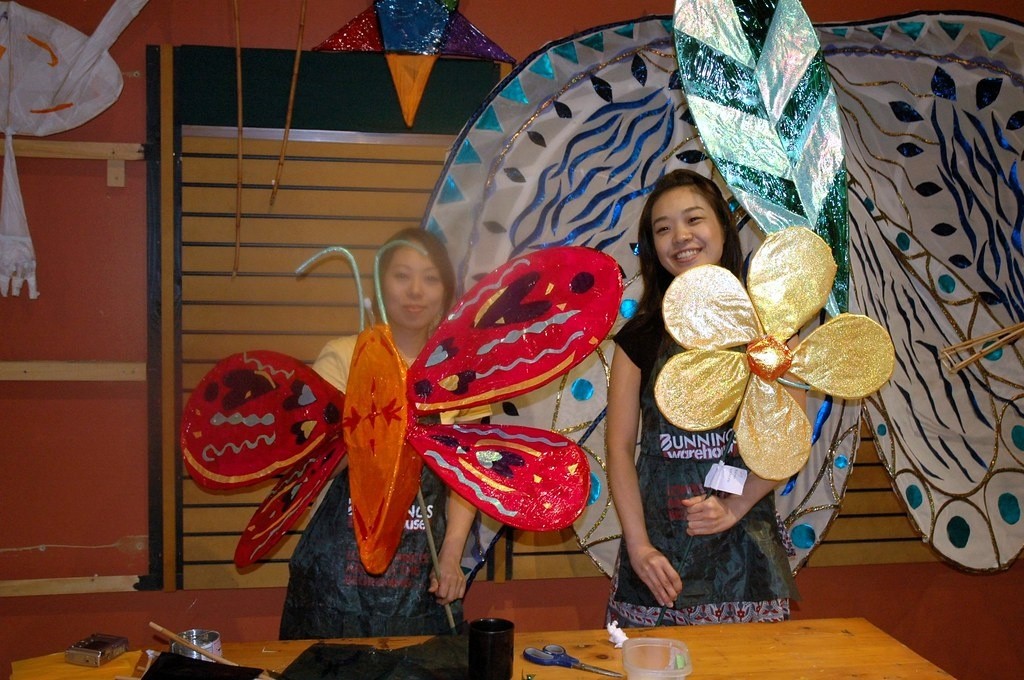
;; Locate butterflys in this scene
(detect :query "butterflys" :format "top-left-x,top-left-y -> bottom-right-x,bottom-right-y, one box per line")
180,239 -> 625,574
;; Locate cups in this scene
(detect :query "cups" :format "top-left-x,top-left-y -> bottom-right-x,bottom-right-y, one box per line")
468,617 -> 516,680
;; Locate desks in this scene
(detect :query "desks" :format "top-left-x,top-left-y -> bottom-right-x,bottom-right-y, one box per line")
6,616 -> 958,680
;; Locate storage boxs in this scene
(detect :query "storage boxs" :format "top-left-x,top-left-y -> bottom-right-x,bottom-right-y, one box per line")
622,638 -> 693,680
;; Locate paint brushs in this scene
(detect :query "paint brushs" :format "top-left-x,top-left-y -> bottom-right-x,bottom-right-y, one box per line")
149,622 -> 275,679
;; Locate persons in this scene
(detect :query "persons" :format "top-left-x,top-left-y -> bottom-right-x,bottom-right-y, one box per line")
607,169 -> 807,628
279,227 -> 492,640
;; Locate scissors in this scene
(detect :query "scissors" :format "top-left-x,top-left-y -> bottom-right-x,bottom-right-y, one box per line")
523,644 -> 622,678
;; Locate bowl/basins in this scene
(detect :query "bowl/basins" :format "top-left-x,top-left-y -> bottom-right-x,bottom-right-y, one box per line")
622,637 -> 692,680
